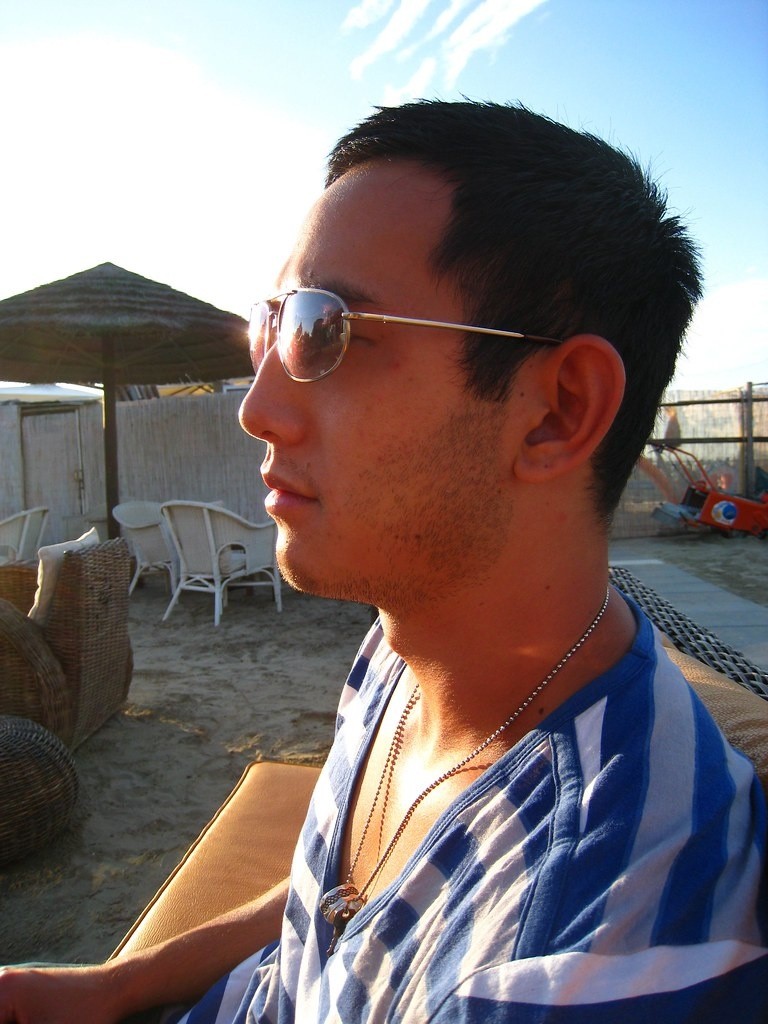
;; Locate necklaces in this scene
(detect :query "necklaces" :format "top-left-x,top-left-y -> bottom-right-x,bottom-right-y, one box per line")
319,580 -> 611,960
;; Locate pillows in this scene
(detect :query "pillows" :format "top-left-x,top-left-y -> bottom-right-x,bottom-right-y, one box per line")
26,526 -> 100,629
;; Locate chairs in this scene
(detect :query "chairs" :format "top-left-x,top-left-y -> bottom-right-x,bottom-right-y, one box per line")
0,505 -> 49,568
160,500 -> 284,627
112,498 -> 178,606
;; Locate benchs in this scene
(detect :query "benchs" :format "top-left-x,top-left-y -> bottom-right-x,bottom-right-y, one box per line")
95,626 -> 765,1024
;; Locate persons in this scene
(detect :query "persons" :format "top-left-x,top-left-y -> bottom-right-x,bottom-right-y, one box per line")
0,99 -> 768,1024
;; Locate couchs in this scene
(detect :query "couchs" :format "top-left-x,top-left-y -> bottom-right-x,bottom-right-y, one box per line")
1,538 -> 135,754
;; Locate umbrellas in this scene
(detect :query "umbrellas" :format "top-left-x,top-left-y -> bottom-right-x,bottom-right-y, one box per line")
0,262 -> 258,544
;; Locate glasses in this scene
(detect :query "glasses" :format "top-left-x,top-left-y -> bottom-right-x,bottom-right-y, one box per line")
247,289 -> 566,383
